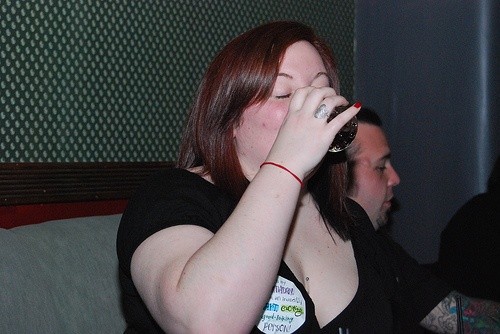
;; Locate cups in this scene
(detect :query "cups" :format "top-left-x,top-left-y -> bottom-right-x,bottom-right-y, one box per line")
326,104 -> 358,153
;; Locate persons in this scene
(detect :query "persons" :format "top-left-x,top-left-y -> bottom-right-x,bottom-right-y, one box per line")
113,19 -> 499,334
334,102 -> 400,235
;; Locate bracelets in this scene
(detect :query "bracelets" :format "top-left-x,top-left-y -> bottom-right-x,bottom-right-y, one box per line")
261,161 -> 304,188
